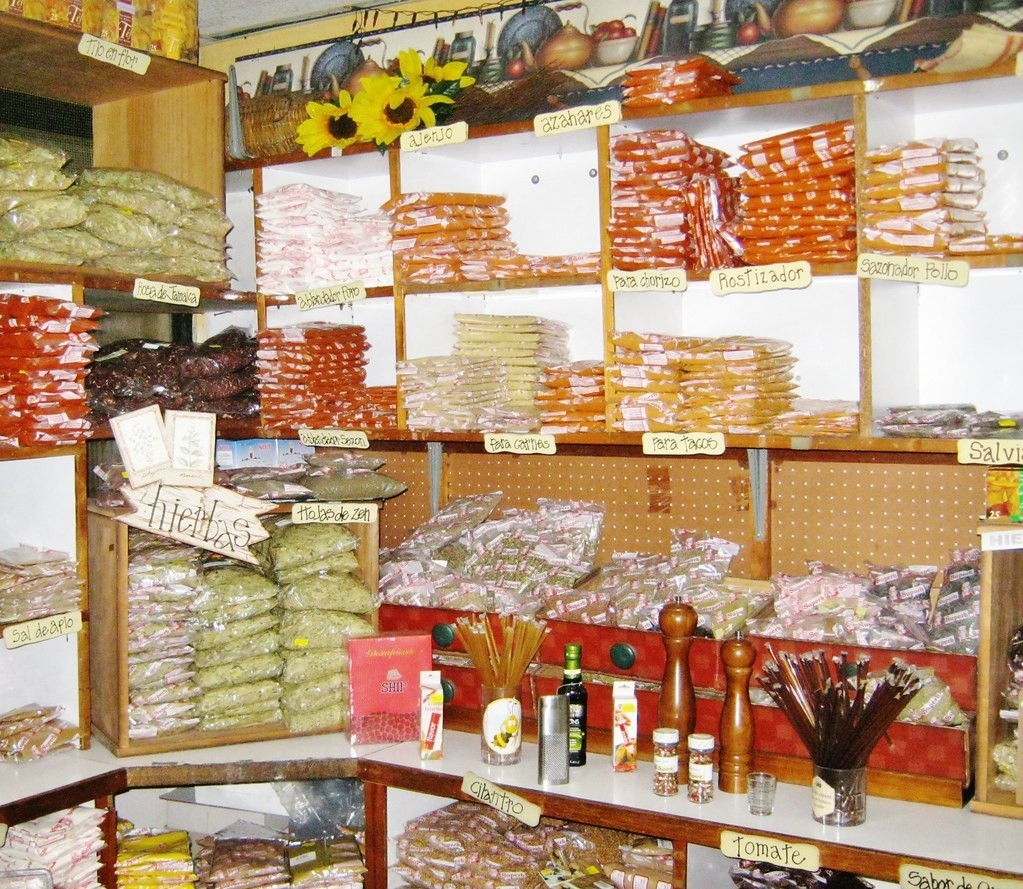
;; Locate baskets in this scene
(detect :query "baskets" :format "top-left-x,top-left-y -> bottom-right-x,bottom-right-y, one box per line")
223,94 -> 324,157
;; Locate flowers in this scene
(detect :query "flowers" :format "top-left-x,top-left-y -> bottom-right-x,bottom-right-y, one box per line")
293,49 -> 478,158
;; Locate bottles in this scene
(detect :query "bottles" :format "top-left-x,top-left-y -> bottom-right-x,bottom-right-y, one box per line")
555,643 -> 587,766
687,732 -> 715,804
652,727 -> 680,796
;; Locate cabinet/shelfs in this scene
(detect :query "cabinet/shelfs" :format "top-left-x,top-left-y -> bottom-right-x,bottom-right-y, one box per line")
0,10 -> 230,753
0,721 -> 1023,889
225,61 -> 1023,460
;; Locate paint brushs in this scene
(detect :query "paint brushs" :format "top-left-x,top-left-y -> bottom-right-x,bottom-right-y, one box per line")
755,640 -> 934,807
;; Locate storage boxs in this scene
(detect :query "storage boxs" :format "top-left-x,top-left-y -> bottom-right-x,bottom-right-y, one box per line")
0,0 -> 201,68
83,483 -> 1023,818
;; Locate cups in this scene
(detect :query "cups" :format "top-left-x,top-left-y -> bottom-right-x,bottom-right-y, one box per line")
479,682 -> 523,767
745,772 -> 776,816
810,761 -> 867,826
